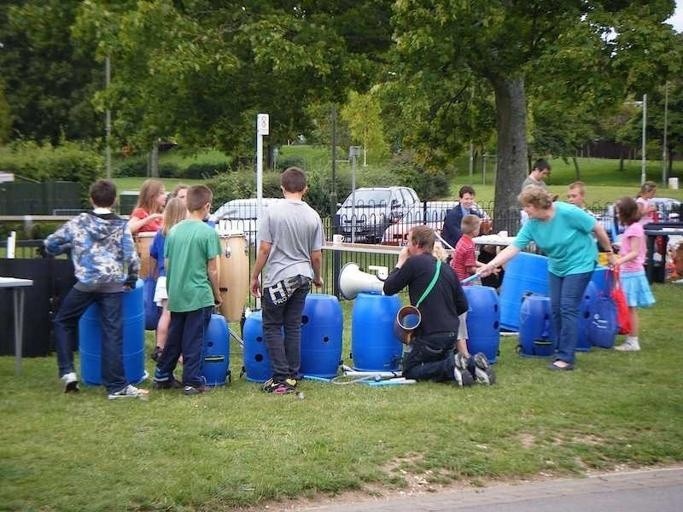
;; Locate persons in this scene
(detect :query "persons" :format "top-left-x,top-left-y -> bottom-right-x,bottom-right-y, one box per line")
43,179 -> 149,400
433,241 -> 471,358
250,166 -> 324,394
128,177 -> 191,361
613,199 -> 656,353
479,188 -> 616,371
151,184 -> 222,396
384,226 -> 495,387
442,159 -> 682,285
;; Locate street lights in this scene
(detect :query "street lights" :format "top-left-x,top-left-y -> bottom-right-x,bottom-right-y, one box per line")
623,94 -> 648,185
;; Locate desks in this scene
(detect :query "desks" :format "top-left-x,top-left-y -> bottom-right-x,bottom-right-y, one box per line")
642,221 -> 683,284
0,276 -> 35,375
472,234 -> 529,288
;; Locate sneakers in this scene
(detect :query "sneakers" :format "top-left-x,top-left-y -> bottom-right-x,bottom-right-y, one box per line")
108,383 -> 149,400
258,378 -> 297,393
613,341 -> 641,350
150,346 -> 210,393
454,352 -> 475,388
549,361 -> 572,371
60,372 -> 80,394
473,352 -> 495,386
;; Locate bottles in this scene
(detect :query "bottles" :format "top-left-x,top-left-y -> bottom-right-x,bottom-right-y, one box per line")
7,231 -> 16,259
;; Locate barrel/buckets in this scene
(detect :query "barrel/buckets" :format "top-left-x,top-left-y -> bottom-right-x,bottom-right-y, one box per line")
202,313 -> 232,387
0,258 -> 49,359
499,252 -> 550,332
76,272 -> 146,387
243,311 -> 273,384
462,285 -> 501,365
51,258 -> 80,353
216,229 -> 249,324
350,289 -> 404,373
300,292 -> 344,380
519,296 -> 557,358
132,230 -> 158,280
575,265 -> 619,353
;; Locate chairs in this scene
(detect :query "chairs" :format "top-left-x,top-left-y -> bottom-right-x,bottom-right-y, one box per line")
608,202 -> 625,244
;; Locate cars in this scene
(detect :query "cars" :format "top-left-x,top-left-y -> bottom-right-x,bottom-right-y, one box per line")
208,198 -> 283,245
381,200 -> 492,250
596,197 -> 683,252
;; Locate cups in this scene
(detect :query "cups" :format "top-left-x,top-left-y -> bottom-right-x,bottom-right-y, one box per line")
499,231 -> 507,241
332,234 -> 343,245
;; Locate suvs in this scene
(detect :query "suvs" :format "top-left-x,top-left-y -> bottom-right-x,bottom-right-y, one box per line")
335,186 -> 420,245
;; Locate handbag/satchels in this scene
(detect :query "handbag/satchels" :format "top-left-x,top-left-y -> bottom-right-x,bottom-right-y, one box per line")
263,275 -> 307,311
587,287 -> 631,349
